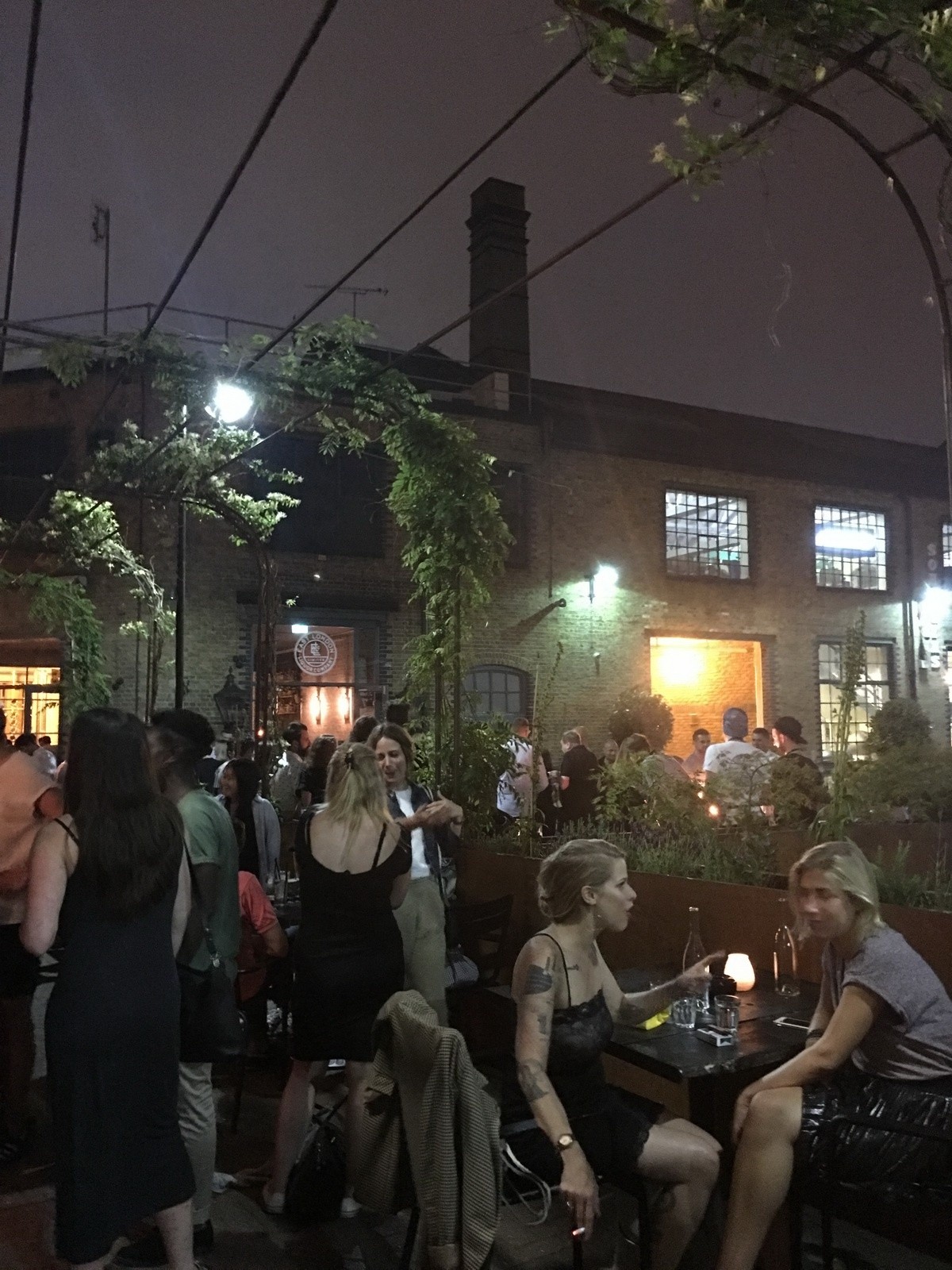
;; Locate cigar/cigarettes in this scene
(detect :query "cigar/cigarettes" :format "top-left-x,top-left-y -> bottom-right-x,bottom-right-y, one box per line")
572,1227 -> 585,1235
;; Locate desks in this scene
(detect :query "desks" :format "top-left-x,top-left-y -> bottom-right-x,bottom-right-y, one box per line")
479,968 -> 822,1270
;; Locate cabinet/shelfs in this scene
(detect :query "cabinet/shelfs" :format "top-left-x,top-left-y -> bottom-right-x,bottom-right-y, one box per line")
267,651 -> 301,729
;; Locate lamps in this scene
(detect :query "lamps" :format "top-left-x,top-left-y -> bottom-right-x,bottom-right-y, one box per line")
344,697 -> 350,723
723,953 -> 755,991
316,698 -> 321,725
584,563 -> 617,605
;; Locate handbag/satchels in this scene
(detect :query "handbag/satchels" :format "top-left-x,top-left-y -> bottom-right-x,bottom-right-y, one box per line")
181,962 -> 245,1063
286,1121 -> 351,1226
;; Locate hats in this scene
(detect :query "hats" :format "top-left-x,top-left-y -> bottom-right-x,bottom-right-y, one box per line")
774,716 -> 809,744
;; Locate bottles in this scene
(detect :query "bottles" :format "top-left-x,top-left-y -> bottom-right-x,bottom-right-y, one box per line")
772,898 -> 801,997
683,907 -> 710,1011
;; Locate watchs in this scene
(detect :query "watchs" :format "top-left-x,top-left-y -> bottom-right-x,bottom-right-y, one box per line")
553,1133 -> 577,1152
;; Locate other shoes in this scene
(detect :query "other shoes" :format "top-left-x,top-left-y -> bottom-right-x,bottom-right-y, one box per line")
0,1125 -> 56,1177
340,1198 -> 359,1218
114,1226 -> 168,1268
261,1183 -> 285,1214
211,1162 -> 273,1194
193,1220 -> 213,1245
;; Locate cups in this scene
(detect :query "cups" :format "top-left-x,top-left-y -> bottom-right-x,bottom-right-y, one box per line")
673,991 -> 697,1029
550,770 -> 560,786
272,870 -> 291,905
714,995 -> 741,1040
649,979 -> 673,1016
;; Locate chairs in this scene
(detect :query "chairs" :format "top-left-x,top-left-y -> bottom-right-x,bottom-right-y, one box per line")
788,1113 -> 952,1270
490,1097 -> 652,1270
457,893 -> 516,1050
178,1008 -> 251,1135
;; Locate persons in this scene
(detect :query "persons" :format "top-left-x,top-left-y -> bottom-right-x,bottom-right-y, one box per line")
0,708 -> 952,1270
